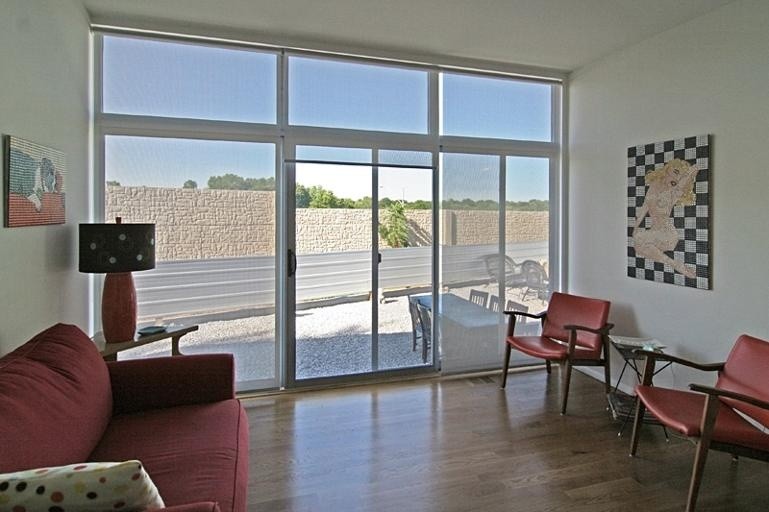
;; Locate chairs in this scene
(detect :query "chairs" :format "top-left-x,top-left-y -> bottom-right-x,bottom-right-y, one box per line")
484,294 -> 504,311
470,287 -> 488,307
503,321 -> 541,338
494,289 -> 615,423
624,332 -> 769,511
508,298 -> 531,325
484,254 -> 548,305
408,293 -> 427,351
417,301 -> 438,360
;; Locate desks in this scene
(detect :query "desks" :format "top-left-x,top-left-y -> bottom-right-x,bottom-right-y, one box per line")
604,332 -> 674,443
412,291 -> 511,370
89,320 -> 199,362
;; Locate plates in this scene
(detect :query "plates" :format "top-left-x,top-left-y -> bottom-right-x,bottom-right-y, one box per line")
138,324 -> 167,336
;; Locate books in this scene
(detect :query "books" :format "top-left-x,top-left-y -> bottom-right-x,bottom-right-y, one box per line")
608,334 -> 667,350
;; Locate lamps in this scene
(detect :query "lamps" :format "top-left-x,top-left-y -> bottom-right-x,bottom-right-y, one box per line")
74,215 -> 157,342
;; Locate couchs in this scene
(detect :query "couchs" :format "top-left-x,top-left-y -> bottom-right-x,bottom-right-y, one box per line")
1,319 -> 250,511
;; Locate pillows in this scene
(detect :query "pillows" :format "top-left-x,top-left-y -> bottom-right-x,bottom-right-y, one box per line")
1,456 -> 171,512
